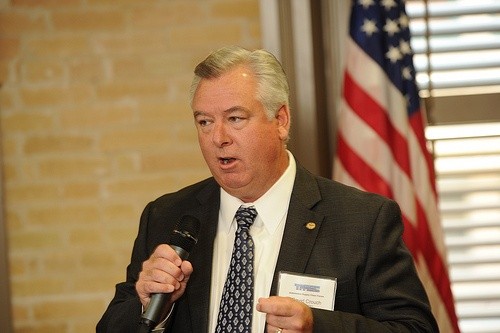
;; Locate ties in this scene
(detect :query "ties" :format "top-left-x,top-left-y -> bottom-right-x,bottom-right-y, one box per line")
215,206 -> 259,333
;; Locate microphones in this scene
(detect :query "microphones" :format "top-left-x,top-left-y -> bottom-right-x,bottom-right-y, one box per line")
138,214 -> 201,333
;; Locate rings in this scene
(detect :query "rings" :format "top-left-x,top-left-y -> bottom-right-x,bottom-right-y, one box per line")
277,329 -> 282,333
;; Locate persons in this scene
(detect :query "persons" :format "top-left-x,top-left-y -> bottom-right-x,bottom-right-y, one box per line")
94,44 -> 440,333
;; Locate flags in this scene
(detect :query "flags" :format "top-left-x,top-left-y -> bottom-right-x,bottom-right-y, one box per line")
331,0 -> 460,333
